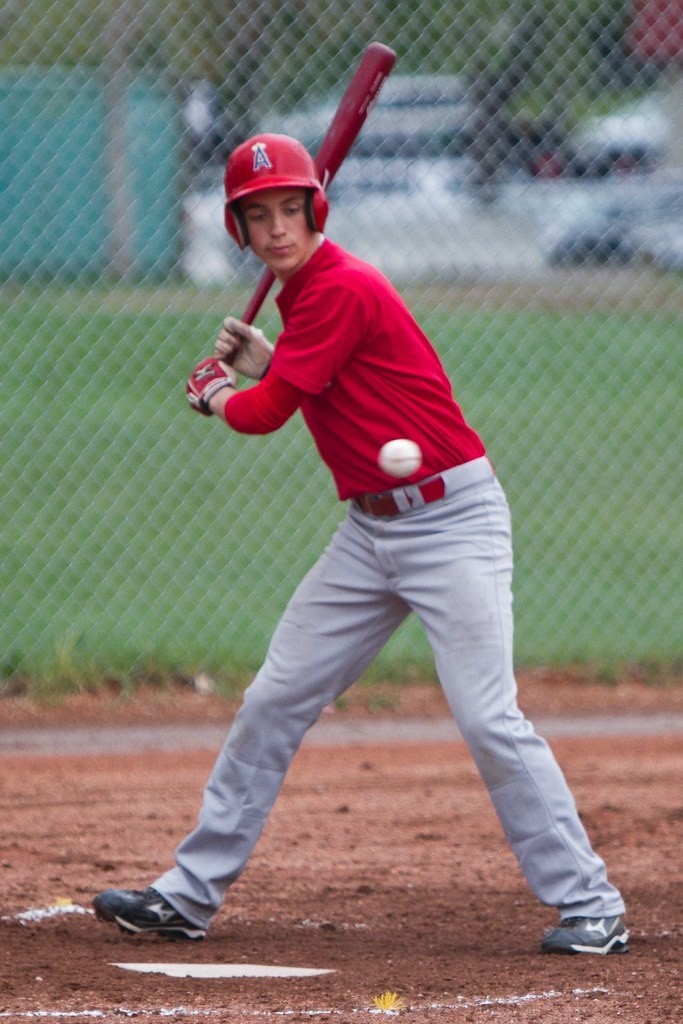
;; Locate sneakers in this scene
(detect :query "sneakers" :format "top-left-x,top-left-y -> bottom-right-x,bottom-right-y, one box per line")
541,916 -> 629,955
91,887 -> 206,941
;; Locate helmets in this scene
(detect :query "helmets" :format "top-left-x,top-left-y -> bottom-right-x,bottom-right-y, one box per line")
224,133 -> 329,252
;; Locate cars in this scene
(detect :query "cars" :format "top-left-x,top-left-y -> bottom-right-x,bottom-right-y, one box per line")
562,87 -> 678,187
334,71 -> 480,185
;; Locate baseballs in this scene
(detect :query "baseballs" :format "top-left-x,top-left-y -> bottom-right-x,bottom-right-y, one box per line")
377,438 -> 423,477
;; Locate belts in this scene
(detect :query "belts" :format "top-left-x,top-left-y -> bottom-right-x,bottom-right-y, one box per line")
350,457 -> 496,520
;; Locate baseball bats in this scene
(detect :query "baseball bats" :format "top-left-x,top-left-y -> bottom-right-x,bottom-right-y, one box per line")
195,40 -> 399,416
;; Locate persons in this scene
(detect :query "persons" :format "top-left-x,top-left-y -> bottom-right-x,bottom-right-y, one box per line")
88,134 -> 634,958
